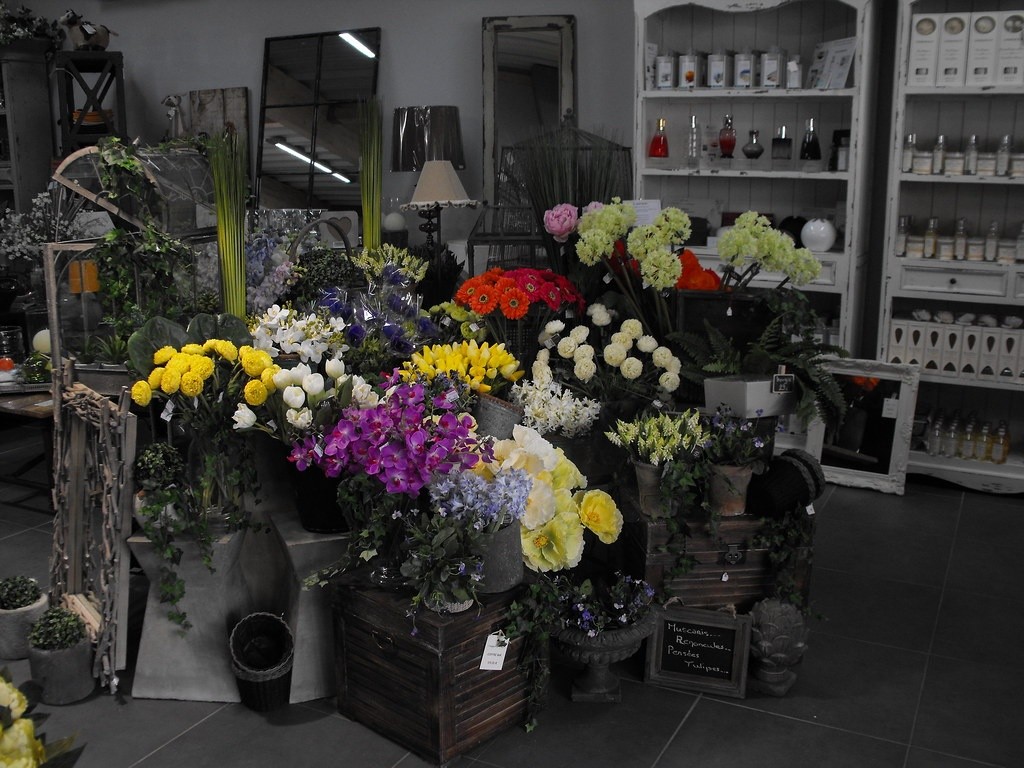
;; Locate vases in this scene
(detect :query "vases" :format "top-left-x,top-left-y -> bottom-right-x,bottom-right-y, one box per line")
704,377 -> 799,420
551,609 -> 656,695
288,455 -> 353,535
634,461 -> 686,519
469,395 -> 524,440
708,464 -> 751,517
126,515 -> 251,703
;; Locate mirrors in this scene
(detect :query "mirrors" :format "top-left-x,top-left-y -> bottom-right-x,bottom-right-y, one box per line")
48,381 -> 138,695
805,355 -> 921,496
251,26 -> 382,257
482,14 -> 576,236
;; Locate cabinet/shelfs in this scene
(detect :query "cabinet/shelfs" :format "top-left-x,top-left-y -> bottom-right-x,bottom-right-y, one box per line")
0,38 -> 54,214
878,0 -> 1024,494
633,0 -> 879,458
56,50 -> 128,156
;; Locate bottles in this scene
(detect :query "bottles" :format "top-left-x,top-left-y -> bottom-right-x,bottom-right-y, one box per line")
655,45 -> 803,88
922,415 -> 1010,464
800,118 -> 822,161
649,119 -> 668,157
719,114 -> 736,158
742,131 -> 764,160
683,116 -> 700,168
895,214 -> 1024,261
903,135 -> 1011,175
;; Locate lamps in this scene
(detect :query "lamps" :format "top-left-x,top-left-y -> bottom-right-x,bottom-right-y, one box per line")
267,138 -> 354,185
392,105 -> 480,307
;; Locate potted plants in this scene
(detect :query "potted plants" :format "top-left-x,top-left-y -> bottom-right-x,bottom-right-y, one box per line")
0,575 -> 48,661
27,605 -> 95,706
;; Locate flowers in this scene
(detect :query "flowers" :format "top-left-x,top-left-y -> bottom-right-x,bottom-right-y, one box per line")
0,2 -> 54,48
120,193 -> 854,734
849,375 -> 877,392
0,179 -> 95,271
0,665 -> 80,768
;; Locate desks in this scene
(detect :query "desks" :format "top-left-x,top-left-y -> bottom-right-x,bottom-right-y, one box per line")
0,392 -> 55,506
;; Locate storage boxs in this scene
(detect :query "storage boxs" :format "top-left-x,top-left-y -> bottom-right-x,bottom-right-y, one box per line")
887,316 -> 1024,379
618,485 -> 816,617
327,565 -> 536,764
906,11 -> 1024,87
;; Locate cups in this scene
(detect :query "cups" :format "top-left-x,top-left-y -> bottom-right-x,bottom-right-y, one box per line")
771,126 -> 792,159
